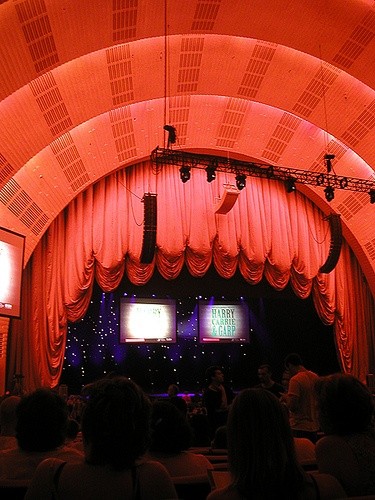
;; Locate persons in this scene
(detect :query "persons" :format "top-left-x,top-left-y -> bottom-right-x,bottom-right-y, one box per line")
279,353 -> 321,444
26,373 -> 178,500
252,365 -> 286,400
313,373 -> 375,500
1,389 -> 71,481
165,383 -> 187,413
207,388 -> 319,500
202,365 -> 237,445
144,395 -> 213,477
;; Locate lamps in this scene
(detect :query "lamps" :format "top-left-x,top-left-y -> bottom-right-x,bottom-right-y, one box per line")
323,185 -> 335,202
368,188 -> 375,204
205,167 -> 217,183
283,176 -> 298,193
179,166 -> 191,183
235,174 -> 247,191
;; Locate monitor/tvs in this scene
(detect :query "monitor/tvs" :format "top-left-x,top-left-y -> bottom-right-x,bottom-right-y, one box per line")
119,297 -> 177,343
198,301 -> 250,343
0,226 -> 26,320
